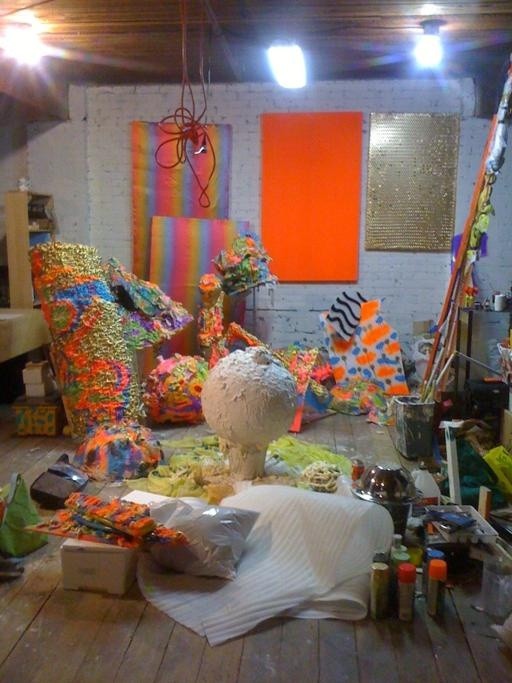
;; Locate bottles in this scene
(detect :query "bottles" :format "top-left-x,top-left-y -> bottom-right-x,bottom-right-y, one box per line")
368,533 -> 448,620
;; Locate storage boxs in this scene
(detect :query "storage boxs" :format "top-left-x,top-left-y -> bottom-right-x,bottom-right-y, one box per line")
22,365 -> 45,383
26,359 -> 51,376
60,536 -> 138,599
25,378 -> 55,397
13,395 -> 63,437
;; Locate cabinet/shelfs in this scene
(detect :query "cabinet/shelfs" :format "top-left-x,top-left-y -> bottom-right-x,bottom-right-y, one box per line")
8,192 -> 58,309
454,306 -> 512,393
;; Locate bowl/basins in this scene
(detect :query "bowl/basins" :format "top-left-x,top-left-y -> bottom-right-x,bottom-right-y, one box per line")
349,460 -> 426,506
497,341 -> 512,357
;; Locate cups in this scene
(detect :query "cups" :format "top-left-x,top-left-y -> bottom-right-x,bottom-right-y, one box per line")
494,294 -> 507,311
480,555 -> 512,617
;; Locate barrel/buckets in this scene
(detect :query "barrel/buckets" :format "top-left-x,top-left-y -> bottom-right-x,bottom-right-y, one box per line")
409,464 -> 442,522
